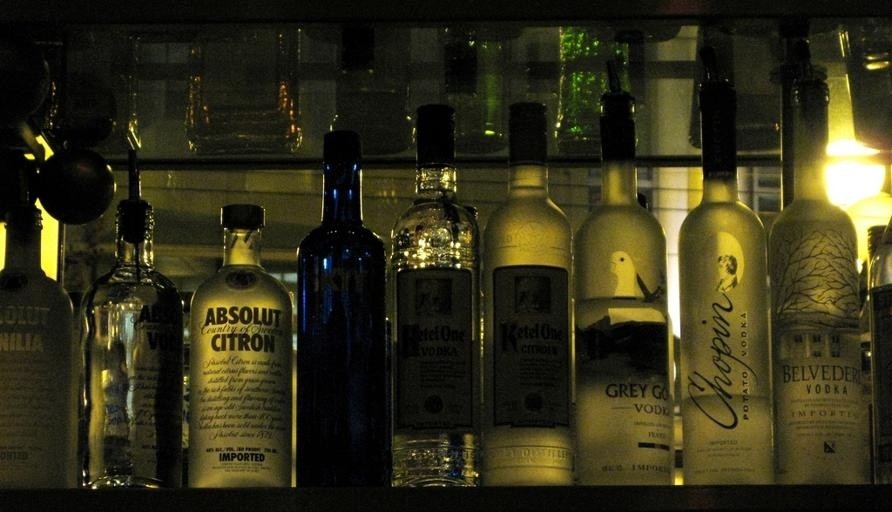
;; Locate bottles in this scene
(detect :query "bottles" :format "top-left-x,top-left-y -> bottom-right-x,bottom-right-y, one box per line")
680,77 -> 768,483
577,90 -> 677,486
398,102 -> 483,489
867,221 -> 891,484
77,197 -> 185,488
768,66 -> 868,482
0,208 -> 80,490
181,200 -> 292,490
296,131 -> 392,488
36,4 -> 892,161
483,99 -> 577,489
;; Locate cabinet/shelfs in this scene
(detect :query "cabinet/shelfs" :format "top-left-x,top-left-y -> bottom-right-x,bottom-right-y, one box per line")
0,0 -> 891,510
